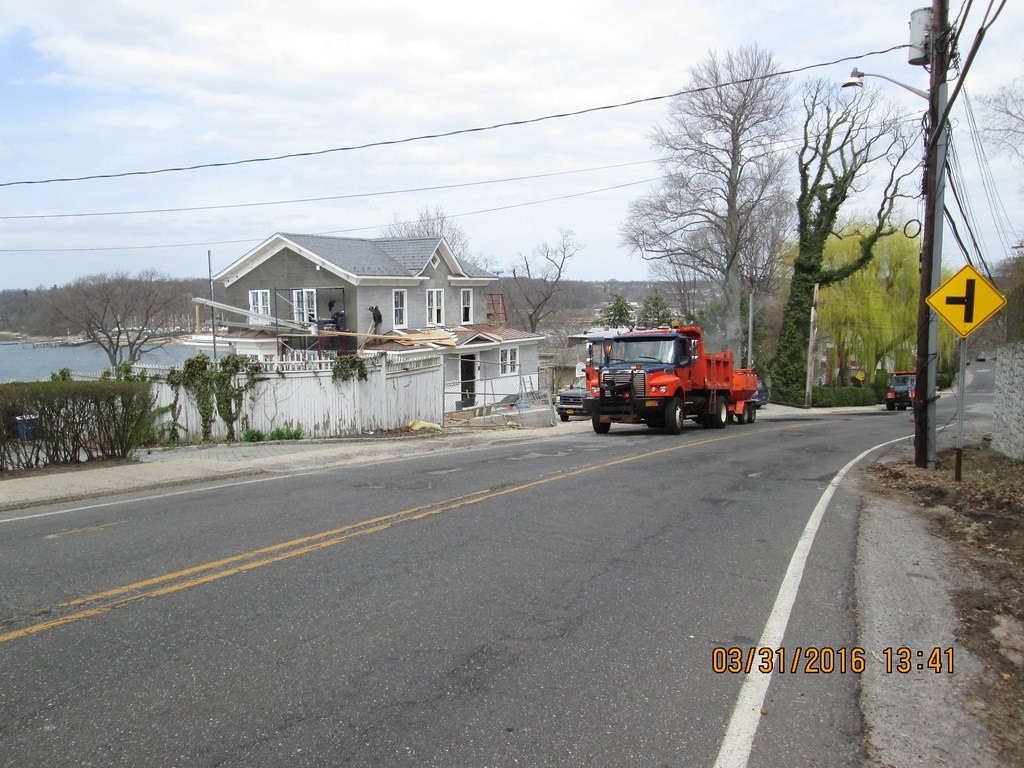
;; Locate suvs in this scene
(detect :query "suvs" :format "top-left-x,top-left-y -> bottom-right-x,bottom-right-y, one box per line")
556,376 -> 594,422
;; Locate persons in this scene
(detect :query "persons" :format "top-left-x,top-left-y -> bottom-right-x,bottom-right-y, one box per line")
332,310 -> 344,330
368,305 -> 382,345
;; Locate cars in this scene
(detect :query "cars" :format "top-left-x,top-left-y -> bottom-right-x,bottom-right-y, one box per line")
756,380 -> 769,409
975,355 -> 986,361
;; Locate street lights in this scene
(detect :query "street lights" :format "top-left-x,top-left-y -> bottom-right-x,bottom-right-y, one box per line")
840,70 -> 954,471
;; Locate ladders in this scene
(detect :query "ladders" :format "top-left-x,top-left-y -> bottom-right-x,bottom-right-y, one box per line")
523,376 -> 534,392
192,296 -> 310,332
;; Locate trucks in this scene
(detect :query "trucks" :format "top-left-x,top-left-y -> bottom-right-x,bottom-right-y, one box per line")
885,371 -> 918,412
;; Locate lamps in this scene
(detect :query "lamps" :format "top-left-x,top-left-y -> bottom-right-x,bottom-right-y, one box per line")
315,264 -> 321,272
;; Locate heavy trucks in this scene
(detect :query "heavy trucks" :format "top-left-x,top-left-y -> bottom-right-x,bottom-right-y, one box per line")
582,319 -> 758,435
